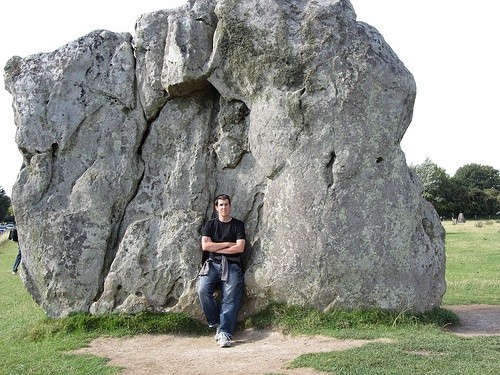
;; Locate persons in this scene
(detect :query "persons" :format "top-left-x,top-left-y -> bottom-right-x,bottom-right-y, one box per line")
198,193 -> 246,348
12,246 -> 21,275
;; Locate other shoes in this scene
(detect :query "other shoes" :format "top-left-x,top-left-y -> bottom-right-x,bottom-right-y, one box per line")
215,326 -> 223,340
219,333 -> 232,348
12,272 -> 17,275
